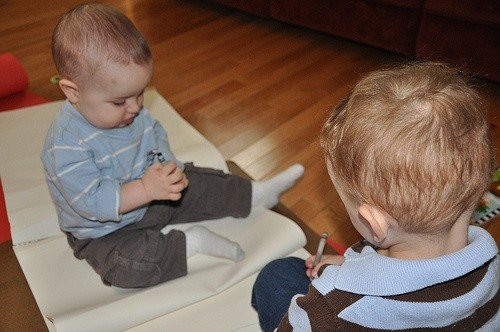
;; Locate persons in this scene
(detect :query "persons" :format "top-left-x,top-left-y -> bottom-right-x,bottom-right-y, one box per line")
250,62 -> 500,332
40,3 -> 304,289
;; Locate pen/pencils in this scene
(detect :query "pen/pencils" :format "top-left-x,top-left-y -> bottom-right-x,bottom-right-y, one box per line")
311,231 -> 328,280
157,153 -> 166,166
